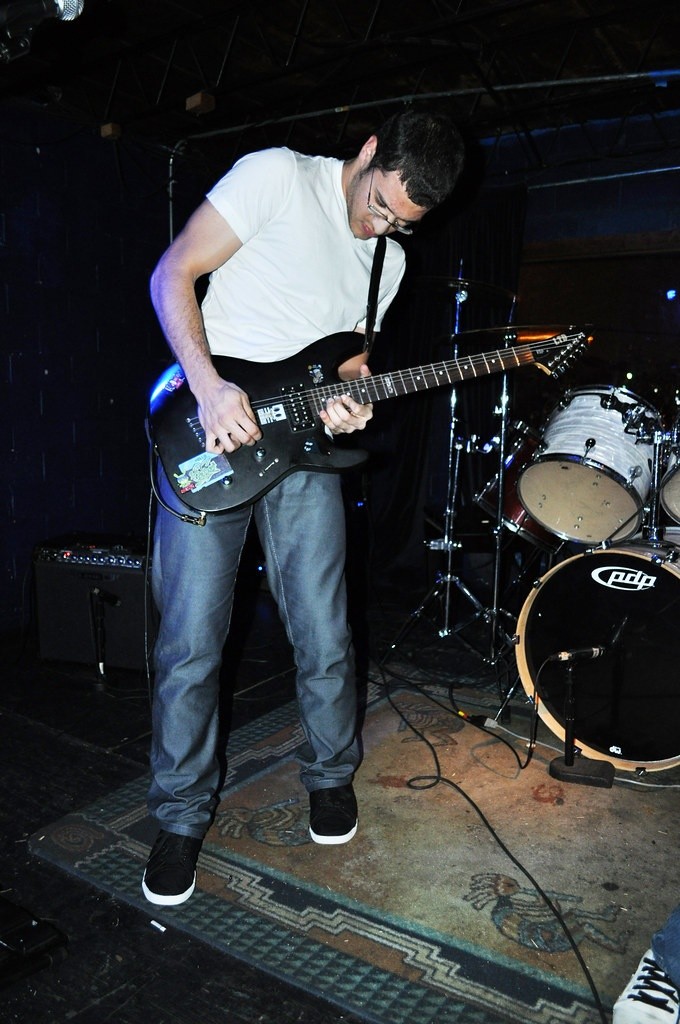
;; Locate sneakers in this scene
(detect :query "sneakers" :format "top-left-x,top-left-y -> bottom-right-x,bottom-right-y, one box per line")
141,828 -> 201,906
609,948 -> 680,1024
307,783 -> 360,843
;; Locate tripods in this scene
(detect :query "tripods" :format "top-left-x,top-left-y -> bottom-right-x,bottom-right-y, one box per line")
378,283 -> 518,725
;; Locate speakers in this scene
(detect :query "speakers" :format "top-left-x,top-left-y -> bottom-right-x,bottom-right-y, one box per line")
34,531 -> 160,673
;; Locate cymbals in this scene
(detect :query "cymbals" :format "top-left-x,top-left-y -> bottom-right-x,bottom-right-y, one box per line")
449,324 -> 580,350
421,275 -> 503,304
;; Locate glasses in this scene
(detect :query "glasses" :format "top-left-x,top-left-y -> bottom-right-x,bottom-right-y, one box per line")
365,161 -> 414,236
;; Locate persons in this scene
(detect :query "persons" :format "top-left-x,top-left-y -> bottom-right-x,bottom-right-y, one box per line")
611,902 -> 680,1024
143,113 -> 461,910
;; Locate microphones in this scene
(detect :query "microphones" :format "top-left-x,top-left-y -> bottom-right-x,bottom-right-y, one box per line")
558,647 -> 604,661
0,0 -> 84,27
90,587 -> 122,608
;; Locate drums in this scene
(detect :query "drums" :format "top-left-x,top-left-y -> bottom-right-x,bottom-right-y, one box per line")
515,382 -> 665,545
659,409 -> 680,525
510,527 -> 680,776
475,419 -> 567,557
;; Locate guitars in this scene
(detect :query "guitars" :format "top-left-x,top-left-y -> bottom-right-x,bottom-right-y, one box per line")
148,322 -> 596,517
